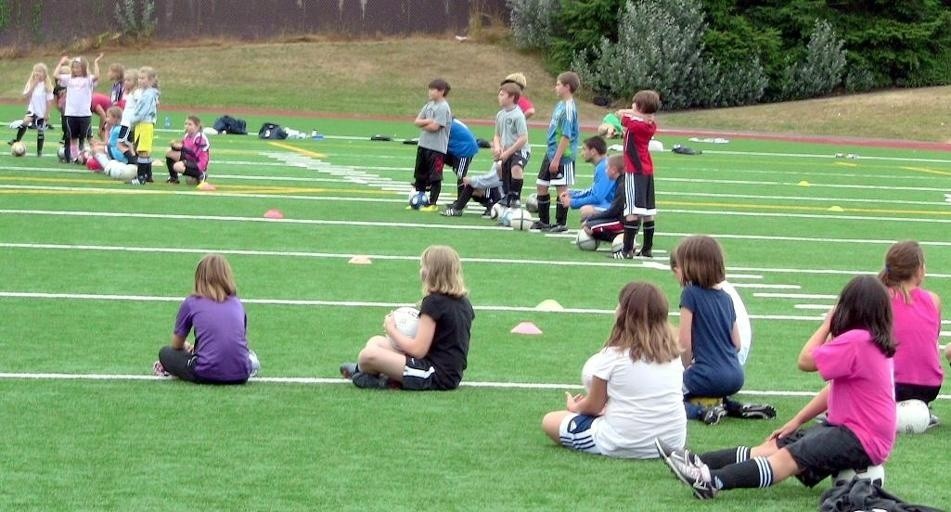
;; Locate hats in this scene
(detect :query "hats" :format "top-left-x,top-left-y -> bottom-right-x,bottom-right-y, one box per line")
500,71 -> 527,89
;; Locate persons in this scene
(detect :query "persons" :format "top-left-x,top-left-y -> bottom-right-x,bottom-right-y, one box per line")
10,51 -> 211,190
339,244 -> 475,391
154,252 -> 261,385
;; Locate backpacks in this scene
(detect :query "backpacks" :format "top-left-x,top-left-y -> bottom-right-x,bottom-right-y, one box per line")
215,114 -> 249,136
258,122 -> 288,140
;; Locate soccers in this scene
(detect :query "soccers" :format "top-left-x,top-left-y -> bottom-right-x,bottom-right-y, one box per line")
895,398 -> 931,434
386,307 -> 422,339
407,192 -> 638,254
10,140 -> 138,179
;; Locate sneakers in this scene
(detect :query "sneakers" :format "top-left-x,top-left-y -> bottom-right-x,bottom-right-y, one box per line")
404,203 -> 439,213
547,223 -> 568,233
128,175 -> 155,186
152,360 -> 176,379
703,401 -> 777,426
652,435 -> 702,467
481,207 -> 491,218
339,361 -> 359,378
510,193 -> 521,207
664,455 -> 718,501
607,248 -> 654,261
166,176 -> 180,184
438,208 -> 464,216
352,372 -> 387,390
197,172 -> 209,184
529,222 -> 550,232
6,137 -> 19,145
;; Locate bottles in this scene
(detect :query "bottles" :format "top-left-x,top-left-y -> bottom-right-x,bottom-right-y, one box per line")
164,116 -> 171,130
